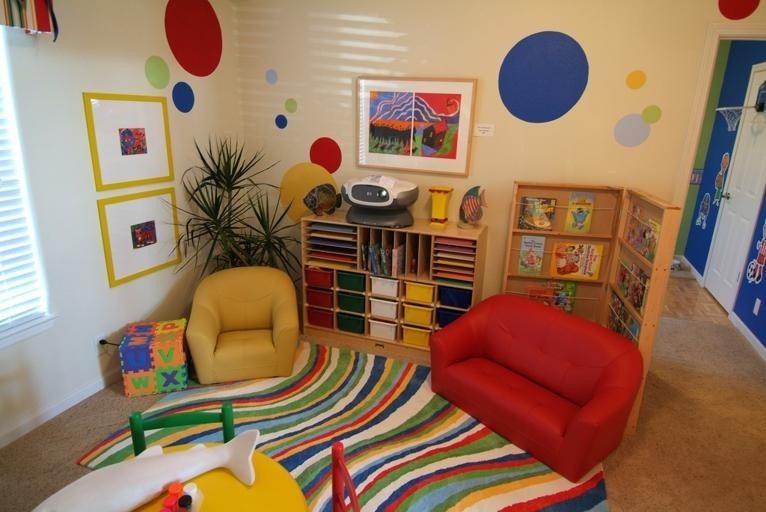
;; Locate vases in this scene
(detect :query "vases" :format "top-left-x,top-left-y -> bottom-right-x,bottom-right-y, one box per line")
427,186 -> 454,227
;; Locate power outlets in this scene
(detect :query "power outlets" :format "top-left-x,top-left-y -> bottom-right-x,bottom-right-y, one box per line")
95,334 -> 109,358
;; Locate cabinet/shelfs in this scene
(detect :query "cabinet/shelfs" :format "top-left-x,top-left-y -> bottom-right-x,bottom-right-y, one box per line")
500,179 -> 621,335
605,189 -> 683,437
299,214 -> 488,353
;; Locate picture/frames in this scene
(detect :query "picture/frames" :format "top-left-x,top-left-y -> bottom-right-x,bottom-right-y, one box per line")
93,185 -> 181,289
81,90 -> 175,193
353,74 -> 478,179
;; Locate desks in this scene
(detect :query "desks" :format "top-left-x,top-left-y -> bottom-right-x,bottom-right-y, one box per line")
121,442 -> 309,512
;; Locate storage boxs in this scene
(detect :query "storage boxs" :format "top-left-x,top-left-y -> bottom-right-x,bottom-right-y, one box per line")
305,267 -> 471,349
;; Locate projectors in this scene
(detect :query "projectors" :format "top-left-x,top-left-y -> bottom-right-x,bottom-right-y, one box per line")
341,176 -> 419,228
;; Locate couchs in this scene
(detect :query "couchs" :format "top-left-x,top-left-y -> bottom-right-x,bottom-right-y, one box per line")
423,291 -> 645,483
181,266 -> 302,385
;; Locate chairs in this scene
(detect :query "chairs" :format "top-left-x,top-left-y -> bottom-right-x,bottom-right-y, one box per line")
127,399 -> 238,462
327,439 -> 362,512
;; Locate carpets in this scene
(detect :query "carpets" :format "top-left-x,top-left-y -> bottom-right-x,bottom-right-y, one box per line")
76,338 -> 610,512
